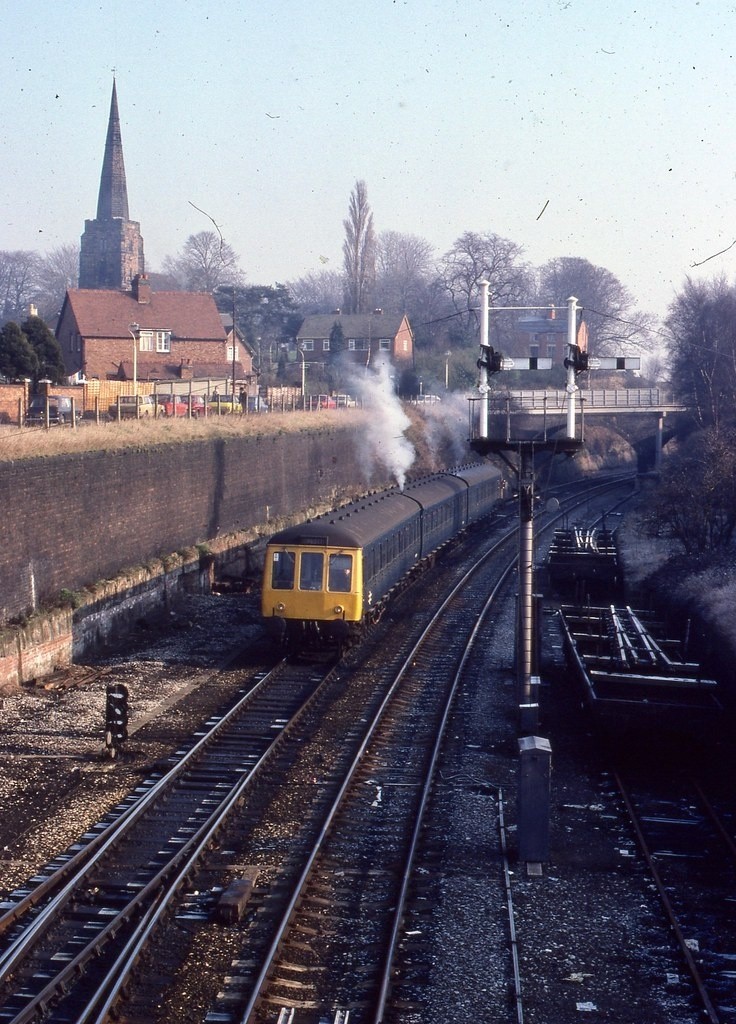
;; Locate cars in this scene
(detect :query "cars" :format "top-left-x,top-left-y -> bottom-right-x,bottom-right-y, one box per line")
25,389 -> 356,426
409,393 -> 441,405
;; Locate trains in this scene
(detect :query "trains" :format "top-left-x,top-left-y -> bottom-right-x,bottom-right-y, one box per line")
259,459 -> 504,663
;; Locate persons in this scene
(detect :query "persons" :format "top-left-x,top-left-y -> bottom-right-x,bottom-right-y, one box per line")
213,385 -> 220,394
238,387 -> 247,415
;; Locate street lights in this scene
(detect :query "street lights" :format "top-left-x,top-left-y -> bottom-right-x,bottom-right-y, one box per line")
128,321 -> 140,395
443,350 -> 452,390
